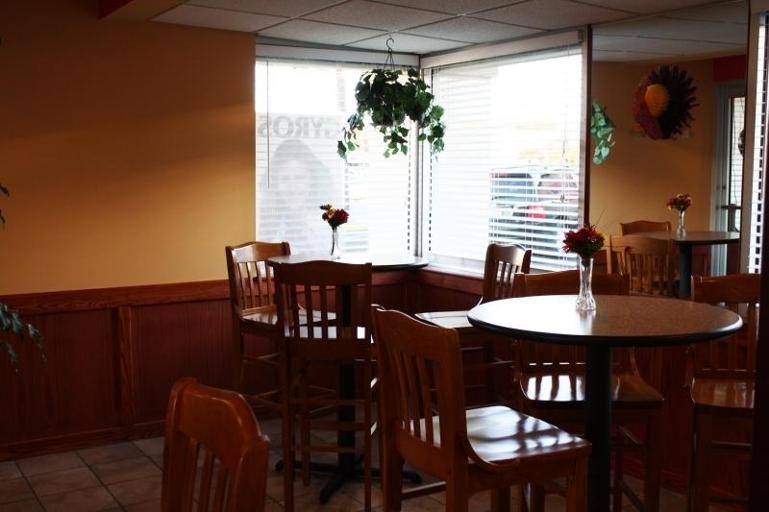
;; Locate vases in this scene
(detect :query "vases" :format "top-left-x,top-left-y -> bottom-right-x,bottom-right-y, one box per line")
676,209 -> 686,234
578,258 -> 597,311
330,229 -> 342,262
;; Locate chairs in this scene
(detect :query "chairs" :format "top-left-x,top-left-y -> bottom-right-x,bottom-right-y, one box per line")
413,244 -> 531,415
158,377 -> 268,511
514,270 -> 665,512
690,273 -> 760,511
369,301 -> 592,512
264,260 -> 378,511
223,240 -> 340,481
603,220 -> 680,301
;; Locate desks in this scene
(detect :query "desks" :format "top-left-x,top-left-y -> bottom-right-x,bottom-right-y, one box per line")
261,250 -> 430,501
623,229 -> 742,299
466,294 -> 744,511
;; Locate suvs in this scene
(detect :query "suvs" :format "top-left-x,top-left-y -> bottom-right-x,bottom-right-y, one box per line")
489,163 -> 581,257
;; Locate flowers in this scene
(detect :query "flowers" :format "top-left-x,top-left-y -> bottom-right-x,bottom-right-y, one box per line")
665,192 -> 693,212
562,224 -> 606,268
319,203 -> 348,229
